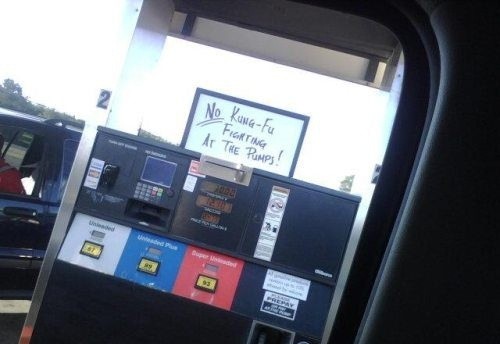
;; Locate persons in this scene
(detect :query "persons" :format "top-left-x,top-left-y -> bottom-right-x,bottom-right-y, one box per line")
0,135 -> 26,195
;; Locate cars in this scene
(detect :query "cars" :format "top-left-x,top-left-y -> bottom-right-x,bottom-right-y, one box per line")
0,106 -> 85,304
